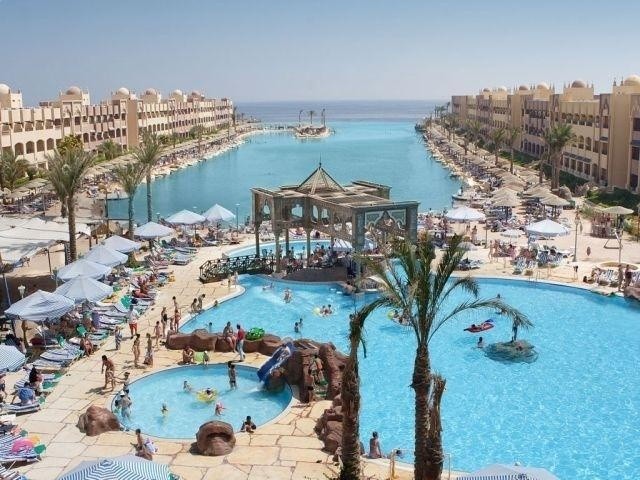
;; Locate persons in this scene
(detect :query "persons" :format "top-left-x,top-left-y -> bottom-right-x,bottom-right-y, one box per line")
424,207 -> 479,244
112,321 -> 257,458
368,431 -> 384,458
0,312 -> 102,405
486,216 -> 550,272
101,275 -> 207,392
617,263 -> 632,292
284,286 -> 333,335
277,242 -> 356,275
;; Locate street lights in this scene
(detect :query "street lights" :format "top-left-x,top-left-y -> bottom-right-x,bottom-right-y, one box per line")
155,213 -> 160,223
43,248 -> 52,275
192,206 -> 197,213
574,217 -> 579,261
78,232 -> 91,250
18,285 -> 25,298
235,204 -> 241,237
87,222 -> 98,244
56,240 -> 68,265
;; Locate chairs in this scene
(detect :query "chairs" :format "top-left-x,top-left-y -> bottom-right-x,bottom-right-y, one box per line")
0,429 -> 37,480
417,204 -> 640,288
3,229 -> 244,416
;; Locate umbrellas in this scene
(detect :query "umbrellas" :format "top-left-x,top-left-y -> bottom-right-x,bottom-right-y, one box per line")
55,455 -> 169,480
456,465 -> 560,480
